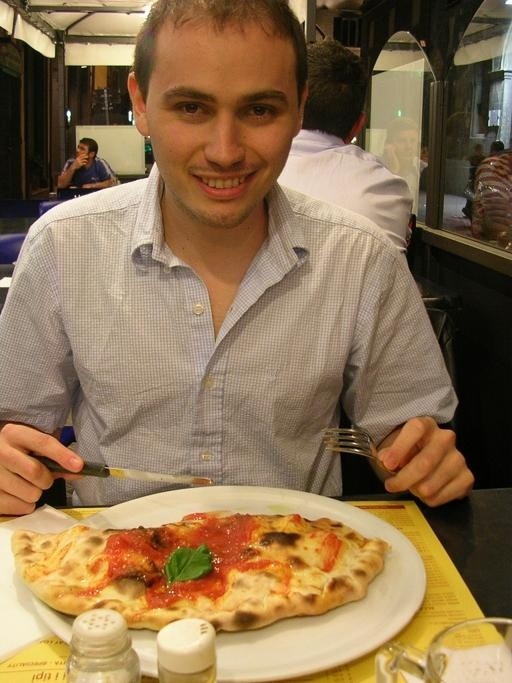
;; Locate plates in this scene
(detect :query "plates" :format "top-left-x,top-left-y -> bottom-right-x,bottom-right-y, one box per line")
31,484 -> 428,683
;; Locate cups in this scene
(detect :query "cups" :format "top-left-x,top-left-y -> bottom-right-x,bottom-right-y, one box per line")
373,617 -> 511,683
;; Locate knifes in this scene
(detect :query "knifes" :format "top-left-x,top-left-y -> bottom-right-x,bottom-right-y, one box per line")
29,454 -> 214,487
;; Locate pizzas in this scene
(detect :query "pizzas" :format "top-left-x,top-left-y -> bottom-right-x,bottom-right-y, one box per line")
11,514 -> 392,634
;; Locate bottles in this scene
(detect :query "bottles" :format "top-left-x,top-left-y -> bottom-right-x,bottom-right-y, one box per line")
65,607 -> 142,683
155,616 -> 218,683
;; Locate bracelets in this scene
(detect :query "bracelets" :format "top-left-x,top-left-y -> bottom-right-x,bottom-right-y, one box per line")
92,183 -> 96,189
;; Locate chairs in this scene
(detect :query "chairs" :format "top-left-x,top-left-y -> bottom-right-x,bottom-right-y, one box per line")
2,185 -> 108,302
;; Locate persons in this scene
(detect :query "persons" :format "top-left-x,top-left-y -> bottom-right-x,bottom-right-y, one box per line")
0,1 -> 480,521
462,141 -> 485,220
489,138 -> 505,155
276,36 -> 415,257
57,137 -> 118,189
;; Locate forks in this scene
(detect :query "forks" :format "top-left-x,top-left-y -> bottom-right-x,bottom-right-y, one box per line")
320,425 -> 401,476
